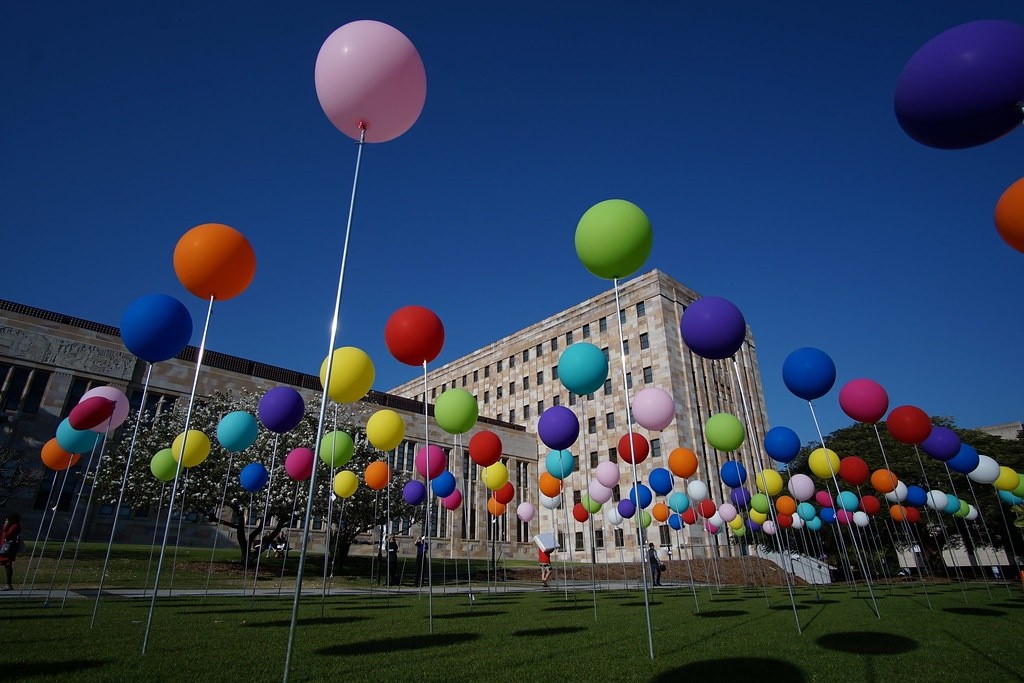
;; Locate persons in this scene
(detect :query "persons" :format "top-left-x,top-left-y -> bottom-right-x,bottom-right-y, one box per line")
539,547 -> 555,587
385,534 -> 398,585
647,543 -> 663,587
0,512 -> 21,590
414,534 -> 429,576
265,530 -> 287,558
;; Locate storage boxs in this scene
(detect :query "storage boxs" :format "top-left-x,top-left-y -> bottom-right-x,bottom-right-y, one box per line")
533,532 -> 560,554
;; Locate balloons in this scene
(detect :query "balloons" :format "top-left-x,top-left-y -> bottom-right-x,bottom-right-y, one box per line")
537,342 -> 751,536
173,223 -> 256,301
517,502 -> 535,522
887,406 -> 1024,504
41,386 -> 129,469
119,294 -> 193,364
149,307 -> 515,516
315,20 -> 427,143
994,177 -> 1024,253
575,199 -> 653,279
748,426 -> 978,534
839,379 -> 889,424
681,297 -> 746,360
894,19 -> 1024,149
782,347 -> 836,401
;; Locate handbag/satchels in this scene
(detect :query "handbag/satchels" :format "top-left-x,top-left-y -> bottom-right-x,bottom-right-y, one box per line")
0,540 -> 19,557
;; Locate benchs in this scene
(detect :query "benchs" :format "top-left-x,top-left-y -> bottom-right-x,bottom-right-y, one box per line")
252,540 -> 293,559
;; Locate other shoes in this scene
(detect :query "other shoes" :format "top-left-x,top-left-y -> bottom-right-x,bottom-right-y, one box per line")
541,579 -> 549,587
0,583 -> 13,591
653,583 -> 658,586
657,582 -> 662,586
